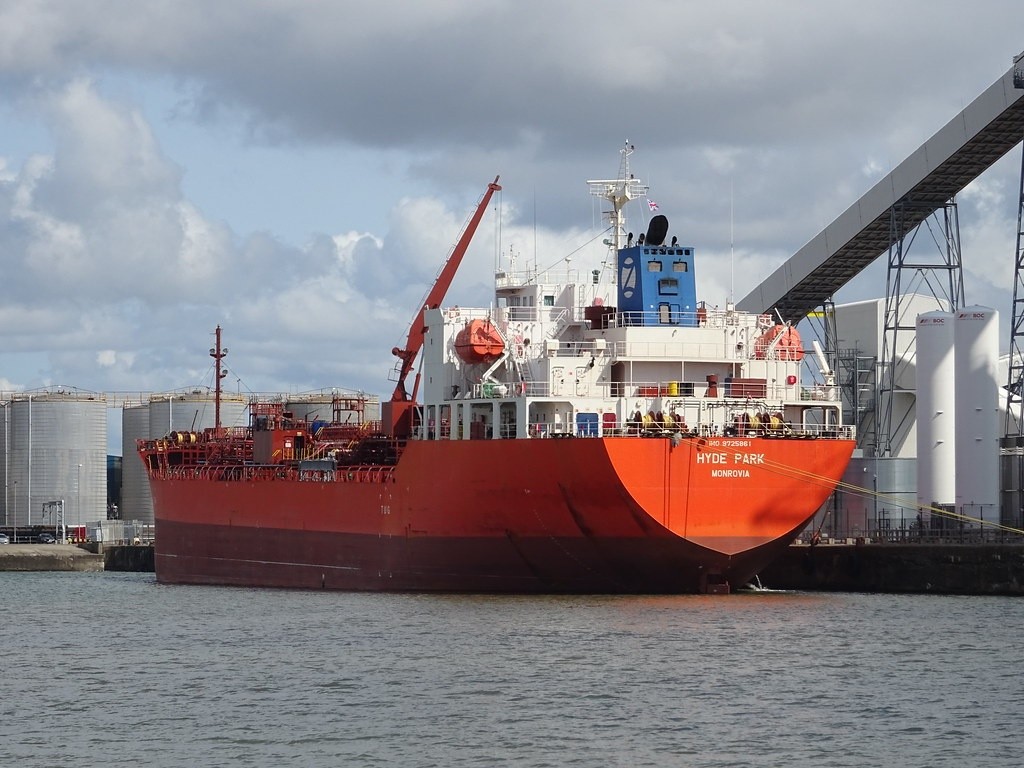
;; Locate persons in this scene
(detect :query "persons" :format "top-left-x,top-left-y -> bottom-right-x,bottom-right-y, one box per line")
724,374 -> 733,396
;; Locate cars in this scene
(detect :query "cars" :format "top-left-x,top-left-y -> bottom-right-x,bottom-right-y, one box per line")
0,533 -> 10,545
37,533 -> 55,544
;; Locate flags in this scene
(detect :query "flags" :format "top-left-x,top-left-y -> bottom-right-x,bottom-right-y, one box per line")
647,198 -> 658,210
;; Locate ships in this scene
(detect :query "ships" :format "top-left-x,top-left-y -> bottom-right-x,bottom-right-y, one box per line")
135,137 -> 858,597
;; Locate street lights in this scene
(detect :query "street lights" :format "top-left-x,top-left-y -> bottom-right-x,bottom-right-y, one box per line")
74,463 -> 82,544
13,480 -> 18,543
